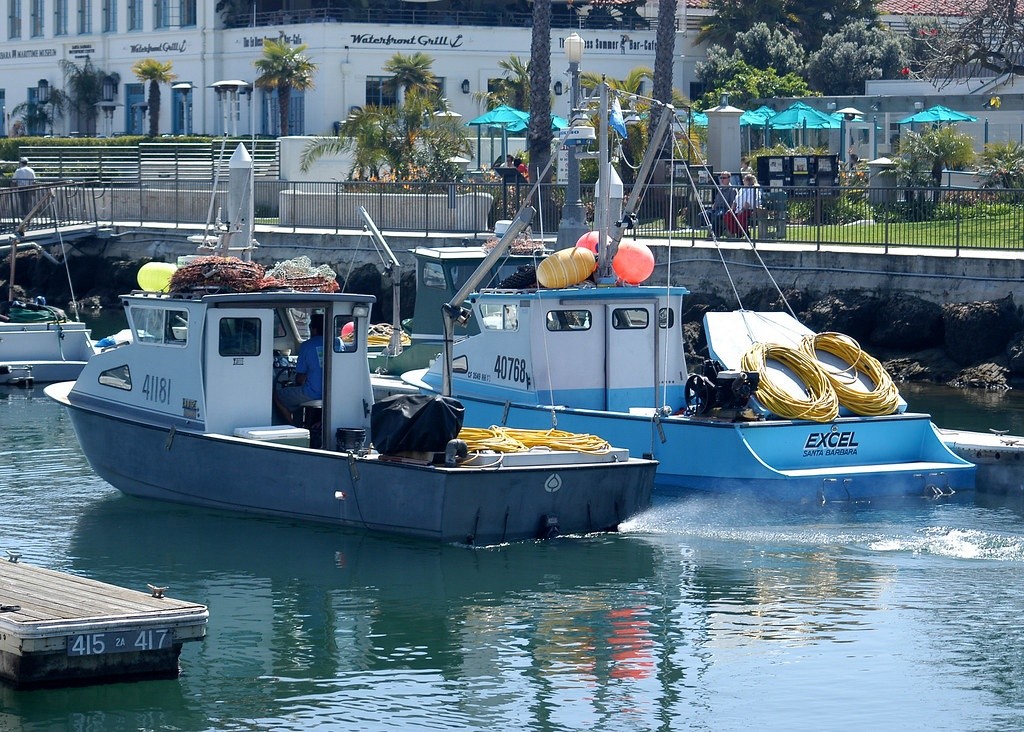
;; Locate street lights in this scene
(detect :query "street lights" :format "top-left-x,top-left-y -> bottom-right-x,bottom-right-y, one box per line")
552,29 -> 591,251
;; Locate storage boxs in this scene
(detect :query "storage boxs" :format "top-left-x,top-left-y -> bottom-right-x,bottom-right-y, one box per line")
233,424 -> 310,449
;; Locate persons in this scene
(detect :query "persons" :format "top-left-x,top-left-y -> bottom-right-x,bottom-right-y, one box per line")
499,153 -> 529,183
739,157 -> 754,174
12,157 -> 37,231
724,174 -> 762,239
704,170 -> 738,238
273,313 -> 325,425
796,161 -> 805,172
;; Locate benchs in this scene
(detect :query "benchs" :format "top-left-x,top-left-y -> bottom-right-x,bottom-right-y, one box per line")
745,191 -> 788,241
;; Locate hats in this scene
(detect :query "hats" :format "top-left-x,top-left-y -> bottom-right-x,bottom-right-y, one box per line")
21,157 -> 28,162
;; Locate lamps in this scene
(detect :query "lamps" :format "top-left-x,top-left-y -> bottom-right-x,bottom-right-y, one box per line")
828,103 -> 837,110
461,78 -> 470,94
870,101 -> 880,111
554,81 -> 562,95
982,100 -> 994,111
915,102 -> 925,110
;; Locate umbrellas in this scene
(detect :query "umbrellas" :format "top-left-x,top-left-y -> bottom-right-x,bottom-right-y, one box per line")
546,111 -> 571,131
688,111 -> 711,126
464,103 -> 530,164
896,104 -> 982,133
739,101 -> 878,157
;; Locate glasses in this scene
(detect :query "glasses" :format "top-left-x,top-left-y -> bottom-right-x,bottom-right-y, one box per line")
720,177 -> 729,179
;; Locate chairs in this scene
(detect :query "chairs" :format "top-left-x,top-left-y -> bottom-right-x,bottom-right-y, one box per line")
300,399 -> 323,428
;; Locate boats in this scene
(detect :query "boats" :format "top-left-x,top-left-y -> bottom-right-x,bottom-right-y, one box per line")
42,0 -> 662,552
0,186 -> 156,388
366,19 -> 979,507
